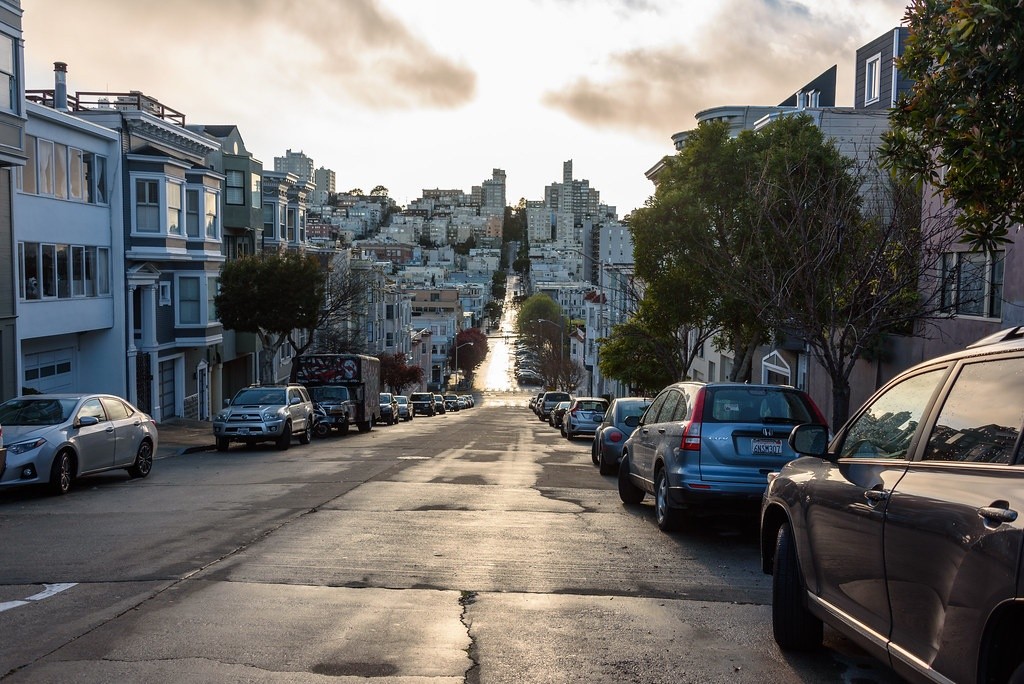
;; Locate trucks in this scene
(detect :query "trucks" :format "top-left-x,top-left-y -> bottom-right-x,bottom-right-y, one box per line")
289,354 -> 381,430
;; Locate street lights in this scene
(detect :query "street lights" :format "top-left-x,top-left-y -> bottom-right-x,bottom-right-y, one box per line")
537,316 -> 564,373
455,342 -> 474,393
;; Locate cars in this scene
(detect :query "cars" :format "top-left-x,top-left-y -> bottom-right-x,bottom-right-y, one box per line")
535,398 -> 543,414
0,393 -> 159,495
516,345 -> 545,384
592,397 -> 655,475
548,402 -> 571,428
528,397 -> 537,412
458,395 -> 474,408
394,396 -> 413,421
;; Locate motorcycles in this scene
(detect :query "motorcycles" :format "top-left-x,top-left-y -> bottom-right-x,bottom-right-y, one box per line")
314,403 -> 331,438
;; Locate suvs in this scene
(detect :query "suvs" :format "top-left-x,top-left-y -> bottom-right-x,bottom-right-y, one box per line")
213,383 -> 314,451
561,397 -> 610,440
408,392 -> 435,417
435,395 -> 445,414
618,382 -> 828,530
535,393 -> 544,413
444,395 -> 459,411
759,326 -> 1023,684
373,393 -> 399,425
538,391 -> 570,421
